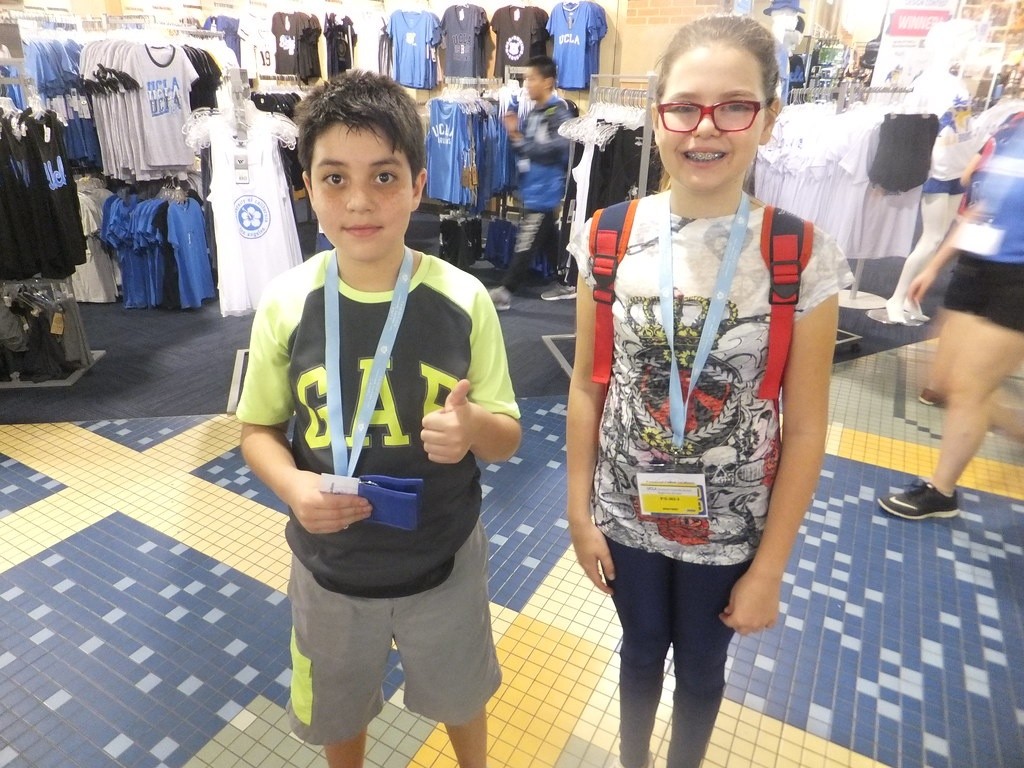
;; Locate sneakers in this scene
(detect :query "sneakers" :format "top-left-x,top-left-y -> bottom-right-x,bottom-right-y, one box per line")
877,479 -> 961,520
541,281 -> 578,301
487,287 -> 512,310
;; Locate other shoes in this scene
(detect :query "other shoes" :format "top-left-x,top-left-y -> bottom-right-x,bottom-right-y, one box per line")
918,388 -> 951,406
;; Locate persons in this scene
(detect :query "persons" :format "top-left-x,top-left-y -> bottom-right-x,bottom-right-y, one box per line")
233,69 -> 523,768
488,55 -> 579,311
877,107 -> 1024,521
887,96 -> 987,326
567,15 -> 855,768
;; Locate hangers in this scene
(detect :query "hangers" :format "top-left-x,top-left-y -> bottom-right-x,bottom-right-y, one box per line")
0,10 -> 307,207
0,279 -> 62,316
425,76 -> 649,152
785,87 -> 909,111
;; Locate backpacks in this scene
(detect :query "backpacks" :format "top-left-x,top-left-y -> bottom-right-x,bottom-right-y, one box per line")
525,99 -> 586,170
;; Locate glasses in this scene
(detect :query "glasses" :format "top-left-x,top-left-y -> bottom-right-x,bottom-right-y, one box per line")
657,100 -> 771,132
